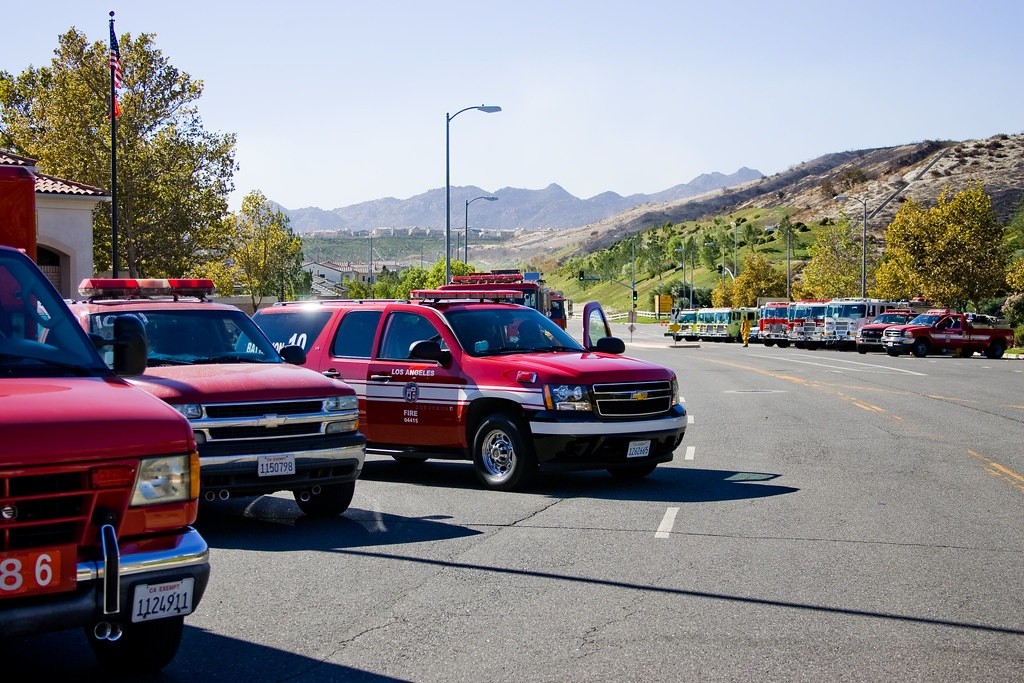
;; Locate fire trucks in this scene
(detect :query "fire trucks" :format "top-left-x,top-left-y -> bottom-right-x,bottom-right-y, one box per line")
422,270 -> 569,333
787,297 -> 833,350
824,296 -> 933,351
662,306 -> 761,344
759,302 -> 791,348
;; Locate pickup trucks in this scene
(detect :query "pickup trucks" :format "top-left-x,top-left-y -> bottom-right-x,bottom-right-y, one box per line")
880,308 -> 1017,358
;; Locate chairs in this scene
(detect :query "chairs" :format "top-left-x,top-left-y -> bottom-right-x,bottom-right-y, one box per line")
170,320 -> 215,355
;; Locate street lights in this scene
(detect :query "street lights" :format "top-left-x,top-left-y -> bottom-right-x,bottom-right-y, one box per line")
731,221 -> 737,280
764,226 -> 790,303
833,196 -> 871,301
674,249 -> 689,309
457,229 -> 471,262
465,195 -> 501,271
704,241 -> 726,309
444,103 -> 505,283
419,239 -> 433,270
370,233 -> 374,285
613,235 -> 636,342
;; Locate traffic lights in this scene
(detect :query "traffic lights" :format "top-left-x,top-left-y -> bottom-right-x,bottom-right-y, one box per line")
717,264 -> 722,274
632,290 -> 638,301
579,270 -> 584,282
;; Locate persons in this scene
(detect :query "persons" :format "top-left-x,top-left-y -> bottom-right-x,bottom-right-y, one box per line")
741,316 -> 751,347
517,321 -> 551,346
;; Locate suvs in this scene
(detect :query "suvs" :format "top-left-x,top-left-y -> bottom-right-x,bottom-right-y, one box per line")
856,309 -> 919,355
0,246 -> 213,683
231,286 -> 688,495
19,278 -> 370,528
